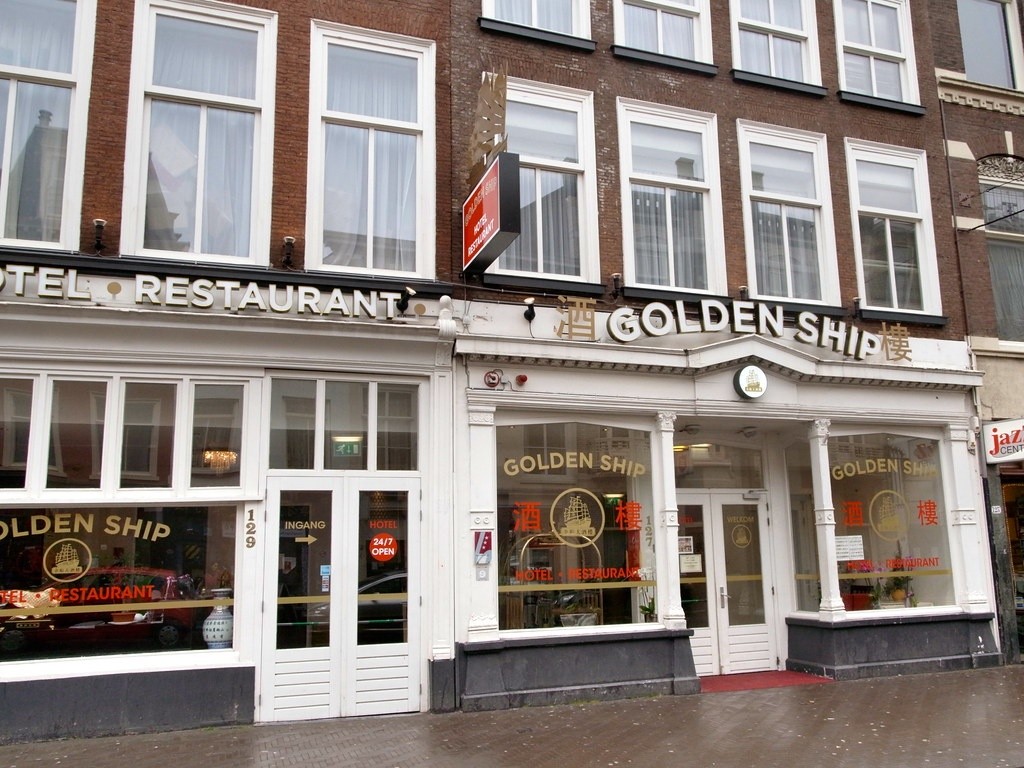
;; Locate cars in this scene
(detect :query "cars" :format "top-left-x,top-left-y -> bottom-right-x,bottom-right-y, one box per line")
307,573 -> 406,634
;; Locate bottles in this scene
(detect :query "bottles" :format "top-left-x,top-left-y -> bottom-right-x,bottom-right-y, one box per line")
203,588 -> 233,649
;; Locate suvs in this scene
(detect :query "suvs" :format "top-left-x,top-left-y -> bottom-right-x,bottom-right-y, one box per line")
1,564 -> 197,657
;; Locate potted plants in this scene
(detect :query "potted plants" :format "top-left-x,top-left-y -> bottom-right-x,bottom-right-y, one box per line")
638,599 -> 657,622
101,548 -> 159,622
557,602 -> 602,626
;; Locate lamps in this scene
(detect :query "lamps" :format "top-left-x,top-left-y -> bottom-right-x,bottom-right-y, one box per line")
203,446 -> 238,476
283,236 -> 296,267
609,273 -> 621,300
684,426 -> 700,435
853,296 -> 861,319
744,427 -> 758,439
397,286 -> 417,316
738,285 -> 749,303
93,219 -> 108,250
734,365 -> 768,399
516,375 -> 528,385
524,297 -> 536,321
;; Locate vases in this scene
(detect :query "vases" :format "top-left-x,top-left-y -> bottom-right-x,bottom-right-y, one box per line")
873,600 -> 905,608
203,587 -> 233,649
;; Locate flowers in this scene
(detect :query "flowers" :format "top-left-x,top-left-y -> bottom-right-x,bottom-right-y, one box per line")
846,553 -> 919,607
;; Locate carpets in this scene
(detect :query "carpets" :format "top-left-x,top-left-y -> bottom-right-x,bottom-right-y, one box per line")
700,671 -> 831,692
